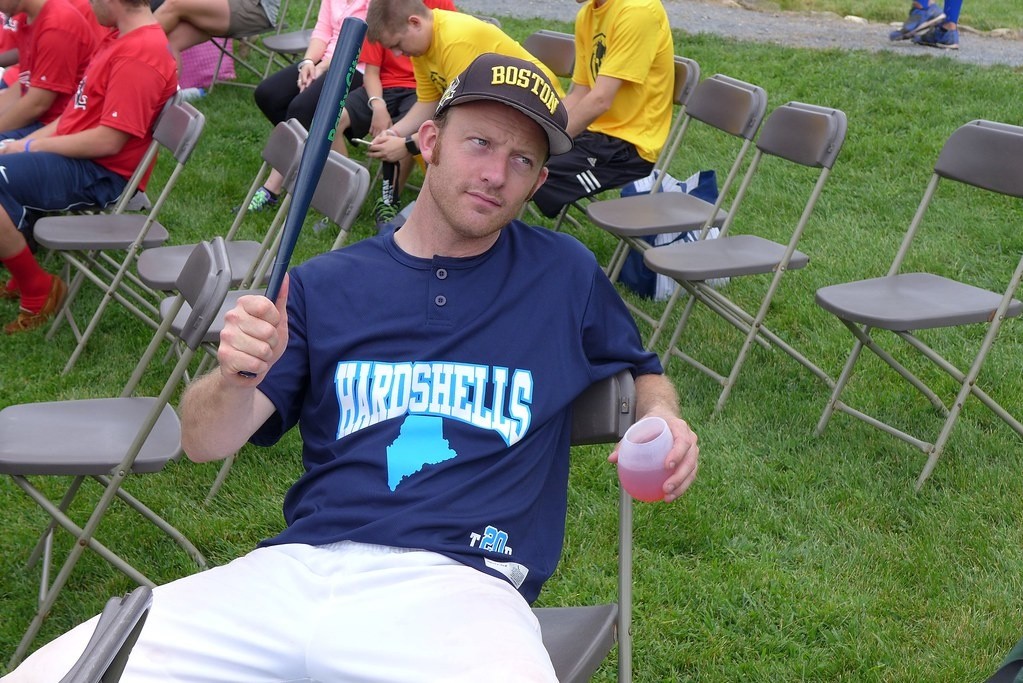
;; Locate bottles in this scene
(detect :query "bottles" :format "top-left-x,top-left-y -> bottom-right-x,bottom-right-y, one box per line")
180,87 -> 208,101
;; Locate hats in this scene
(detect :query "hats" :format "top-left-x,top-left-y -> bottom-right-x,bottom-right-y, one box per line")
432,51 -> 573,156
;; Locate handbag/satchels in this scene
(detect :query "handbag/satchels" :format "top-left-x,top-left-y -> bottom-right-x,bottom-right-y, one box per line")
615,168 -> 730,301
180,38 -> 237,89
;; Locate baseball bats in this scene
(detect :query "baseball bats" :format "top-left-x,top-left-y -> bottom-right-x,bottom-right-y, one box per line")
236,15 -> 369,380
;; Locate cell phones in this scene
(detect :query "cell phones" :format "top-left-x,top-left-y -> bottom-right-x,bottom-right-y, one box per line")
351,139 -> 372,146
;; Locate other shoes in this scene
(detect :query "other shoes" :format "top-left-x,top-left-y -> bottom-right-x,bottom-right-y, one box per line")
0,283 -> 21,299
3,274 -> 68,333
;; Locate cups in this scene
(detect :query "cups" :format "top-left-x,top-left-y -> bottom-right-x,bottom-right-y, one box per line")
616,416 -> 677,502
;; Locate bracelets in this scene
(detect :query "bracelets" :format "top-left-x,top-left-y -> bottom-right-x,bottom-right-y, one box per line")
297,59 -> 313,72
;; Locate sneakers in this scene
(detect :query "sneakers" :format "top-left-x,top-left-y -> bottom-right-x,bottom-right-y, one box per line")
312,217 -> 333,234
231,187 -> 281,216
916,26 -> 960,49
371,199 -> 402,231
890,5 -> 947,41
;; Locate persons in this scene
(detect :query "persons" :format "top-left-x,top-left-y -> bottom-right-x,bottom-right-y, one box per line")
534,0 -> 675,222
244,1 -> 379,215
888,0 -> 963,51
311,1 -> 456,234
153,1 -> 282,76
361,1 -> 567,204
0,0 -> 178,340
0,53 -> 701,683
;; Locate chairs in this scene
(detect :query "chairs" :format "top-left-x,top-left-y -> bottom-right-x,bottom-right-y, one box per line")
0,0 -> 1023,683
